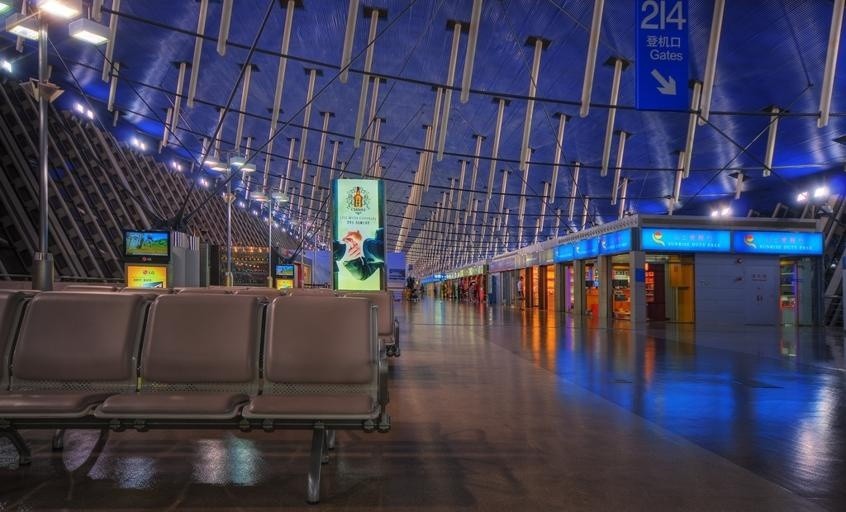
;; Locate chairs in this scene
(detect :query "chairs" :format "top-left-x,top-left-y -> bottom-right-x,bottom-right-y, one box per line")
0,286 -> 401,506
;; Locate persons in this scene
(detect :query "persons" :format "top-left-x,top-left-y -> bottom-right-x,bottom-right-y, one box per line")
411,289 -> 420,301
518,276 -> 526,311
420,284 -> 424,300
433,283 -> 437,298
334,230 -> 384,286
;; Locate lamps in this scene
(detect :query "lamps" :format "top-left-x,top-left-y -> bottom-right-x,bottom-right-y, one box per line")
69,19 -> 111,46
0,57 -> 289,202
5,12 -> 39,40
37,1 -> 83,19
